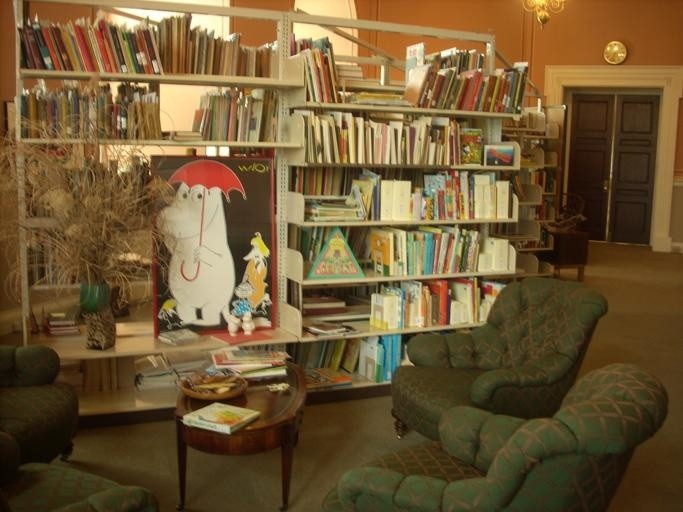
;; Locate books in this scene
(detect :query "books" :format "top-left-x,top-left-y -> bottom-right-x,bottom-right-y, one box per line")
17,13 -> 278,142
293,224 -> 508,280
292,282 -> 508,340
292,335 -> 402,389
293,37 -> 529,112
55,345 -> 292,398
293,167 -> 514,221
292,109 -> 514,165
183,402 -> 261,434
44,311 -> 81,336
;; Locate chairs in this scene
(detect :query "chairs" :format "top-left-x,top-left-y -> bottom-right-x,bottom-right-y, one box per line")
0,341 -> 160,510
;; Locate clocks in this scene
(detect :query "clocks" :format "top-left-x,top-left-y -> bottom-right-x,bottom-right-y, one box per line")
603,41 -> 627,65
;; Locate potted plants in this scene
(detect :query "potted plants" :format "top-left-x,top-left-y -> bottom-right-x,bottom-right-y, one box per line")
11,110 -> 178,350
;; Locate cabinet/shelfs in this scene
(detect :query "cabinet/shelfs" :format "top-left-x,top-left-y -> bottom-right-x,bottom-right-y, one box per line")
503,99 -> 566,274
13,10 -> 287,424
290,9 -> 513,393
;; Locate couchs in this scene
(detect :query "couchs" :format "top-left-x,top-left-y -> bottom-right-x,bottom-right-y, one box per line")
335,362 -> 667,508
391,273 -> 608,442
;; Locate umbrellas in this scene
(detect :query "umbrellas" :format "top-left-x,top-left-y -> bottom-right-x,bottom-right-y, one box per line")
168,160 -> 247,281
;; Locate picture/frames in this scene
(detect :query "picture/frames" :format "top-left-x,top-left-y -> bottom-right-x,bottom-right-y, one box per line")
150,153 -> 276,333
